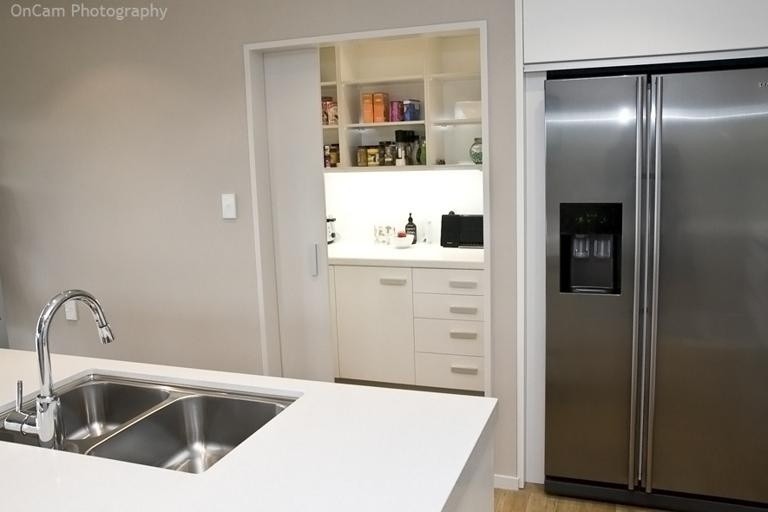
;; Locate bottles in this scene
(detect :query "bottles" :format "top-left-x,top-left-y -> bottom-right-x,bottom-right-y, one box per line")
404,212 -> 417,245
356,129 -> 426,166
467,136 -> 485,164
324,142 -> 339,168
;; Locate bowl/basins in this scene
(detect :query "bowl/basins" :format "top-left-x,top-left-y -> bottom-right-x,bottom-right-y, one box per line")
387,234 -> 414,248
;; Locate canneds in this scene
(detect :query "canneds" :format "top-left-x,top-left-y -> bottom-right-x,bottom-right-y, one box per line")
325,145 -> 330,167
390,101 -> 404,122
322,96 -> 333,126
356,140 -> 395,166
330,143 -> 339,167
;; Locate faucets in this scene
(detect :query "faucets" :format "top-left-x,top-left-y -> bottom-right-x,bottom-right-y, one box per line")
34,287 -> 116,448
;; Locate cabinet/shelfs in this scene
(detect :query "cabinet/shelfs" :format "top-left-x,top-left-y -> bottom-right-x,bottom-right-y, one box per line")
428,72 -> 482,170
332,266 -> 483,392
319,42 -> 342,173
339,75 -> 426,172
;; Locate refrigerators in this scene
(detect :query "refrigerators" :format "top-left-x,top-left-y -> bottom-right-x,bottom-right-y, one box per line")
544,67 -> 766,511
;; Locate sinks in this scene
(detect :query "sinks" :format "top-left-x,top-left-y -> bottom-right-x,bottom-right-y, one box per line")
0,365 -> 174,445
82,387 -> 305,477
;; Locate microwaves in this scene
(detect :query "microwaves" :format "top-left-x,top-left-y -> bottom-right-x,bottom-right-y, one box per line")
439,213 -> 483,250
